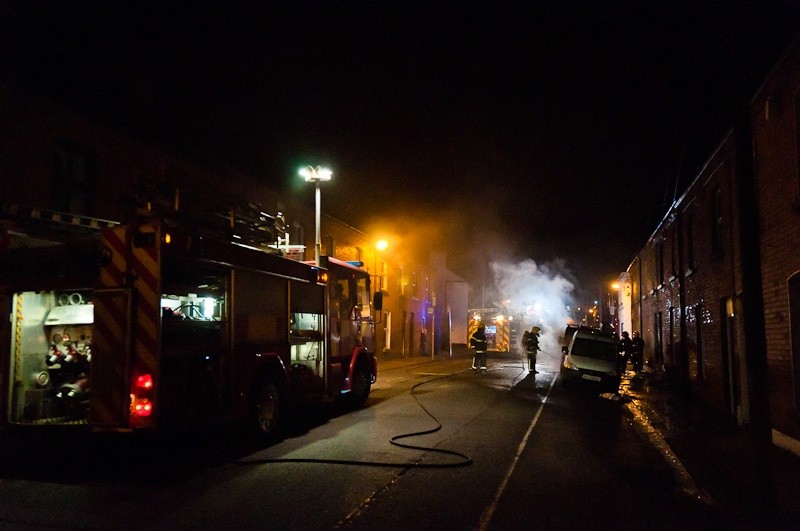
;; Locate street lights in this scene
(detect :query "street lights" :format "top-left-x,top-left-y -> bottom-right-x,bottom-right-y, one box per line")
299,163 -> 332,268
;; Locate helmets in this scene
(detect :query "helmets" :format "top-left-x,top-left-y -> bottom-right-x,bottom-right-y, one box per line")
621,331 -> 628,337
532,327 -> 541,334
478,324 -> 485,328
633,331 -> 639,336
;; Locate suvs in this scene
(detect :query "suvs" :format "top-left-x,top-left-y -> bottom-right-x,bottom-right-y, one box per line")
556,323 -> 624,394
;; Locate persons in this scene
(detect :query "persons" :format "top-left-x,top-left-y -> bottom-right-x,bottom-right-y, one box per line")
469,324 -> 487,375
520,327 -> 542,373
621,330 -> 645,375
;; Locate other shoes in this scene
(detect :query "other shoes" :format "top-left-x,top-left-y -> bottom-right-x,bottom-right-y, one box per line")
530,370 -> 539,373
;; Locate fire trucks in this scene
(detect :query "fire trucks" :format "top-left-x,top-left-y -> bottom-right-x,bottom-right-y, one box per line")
467,306 -> 525,357
0,201 -> 384,491
